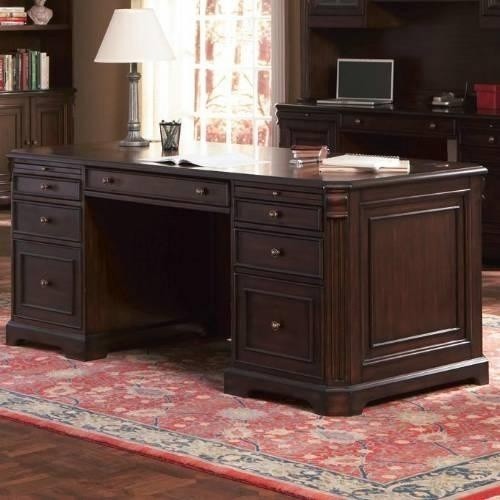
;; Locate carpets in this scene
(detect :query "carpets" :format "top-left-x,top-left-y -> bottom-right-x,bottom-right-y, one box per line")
0,255 -> 500,498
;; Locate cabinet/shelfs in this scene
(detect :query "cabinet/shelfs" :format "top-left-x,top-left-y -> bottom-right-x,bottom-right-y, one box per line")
0,0 -> 74,93
0,95 -> 74,204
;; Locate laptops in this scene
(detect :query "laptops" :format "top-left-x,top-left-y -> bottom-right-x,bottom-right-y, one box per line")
316,58 -> 394,106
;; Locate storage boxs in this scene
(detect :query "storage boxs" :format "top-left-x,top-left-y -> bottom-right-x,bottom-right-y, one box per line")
472,82 -> 500,110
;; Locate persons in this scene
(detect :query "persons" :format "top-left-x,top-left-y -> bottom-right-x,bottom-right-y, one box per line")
27,0 -> 53,25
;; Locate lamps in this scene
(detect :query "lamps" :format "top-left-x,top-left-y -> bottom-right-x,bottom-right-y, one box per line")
92,8 -> 174,147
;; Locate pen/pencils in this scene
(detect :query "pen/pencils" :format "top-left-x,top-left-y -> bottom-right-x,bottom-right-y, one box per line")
162,120 -> 180,151
299,160 -> 322,164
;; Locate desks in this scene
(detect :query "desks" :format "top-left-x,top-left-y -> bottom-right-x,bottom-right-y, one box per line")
4,141 -> 490,420
274,103 -> 499,274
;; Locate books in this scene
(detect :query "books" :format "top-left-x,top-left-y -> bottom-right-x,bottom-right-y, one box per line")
137,152 -> 272,168
0,6 -> 51,93
317,159 -> 412,174
288,144 -> 329,164
320,152 -> 400,173
